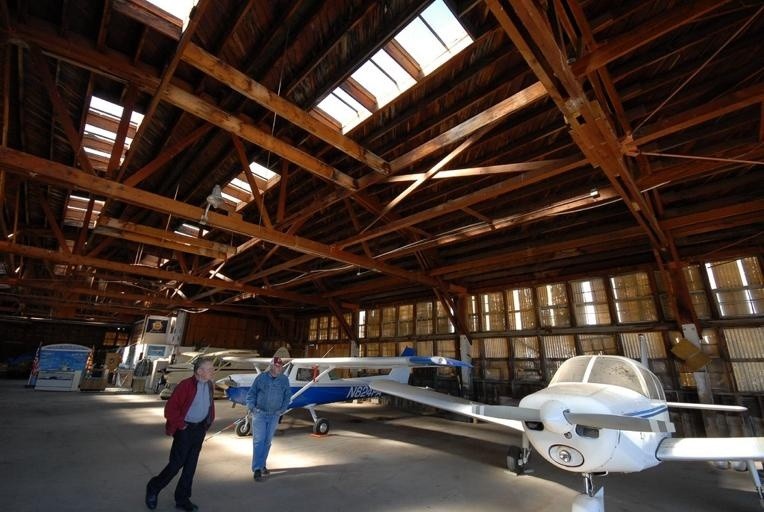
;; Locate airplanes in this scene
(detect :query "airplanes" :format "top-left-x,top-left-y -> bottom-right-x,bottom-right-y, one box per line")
367,334 -> 764,512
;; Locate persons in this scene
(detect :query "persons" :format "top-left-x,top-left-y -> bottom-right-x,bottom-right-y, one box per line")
144,356 -> 217,512
245,354 -> 292,482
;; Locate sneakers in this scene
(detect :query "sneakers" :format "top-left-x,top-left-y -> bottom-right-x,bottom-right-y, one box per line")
254,467 -> 269,481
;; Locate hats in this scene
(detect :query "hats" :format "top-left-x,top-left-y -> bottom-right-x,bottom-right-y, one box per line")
271,357 -> 283,366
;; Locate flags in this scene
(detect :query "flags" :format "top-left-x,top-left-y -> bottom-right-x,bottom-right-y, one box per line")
30,344 -> 40,376
85,348 -> 93,380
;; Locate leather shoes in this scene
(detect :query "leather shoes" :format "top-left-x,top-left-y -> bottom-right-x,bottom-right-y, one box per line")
176,500 -> 199,512
145,483 -> 158,510
